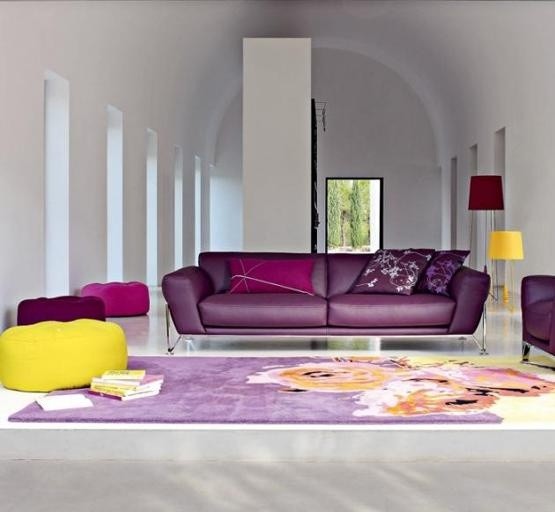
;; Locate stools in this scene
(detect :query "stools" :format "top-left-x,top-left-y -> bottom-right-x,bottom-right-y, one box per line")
1,282 -> 150,394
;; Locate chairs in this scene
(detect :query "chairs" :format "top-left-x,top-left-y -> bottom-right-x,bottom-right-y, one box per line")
521,274 -> 555,361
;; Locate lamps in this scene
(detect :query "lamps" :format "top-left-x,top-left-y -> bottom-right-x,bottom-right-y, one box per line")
468,176 -> 525,314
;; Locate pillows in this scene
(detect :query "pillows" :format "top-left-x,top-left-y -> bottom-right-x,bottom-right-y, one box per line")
228,249 -> 470,295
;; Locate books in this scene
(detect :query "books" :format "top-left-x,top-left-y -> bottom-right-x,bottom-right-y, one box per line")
88,370 -> 163,401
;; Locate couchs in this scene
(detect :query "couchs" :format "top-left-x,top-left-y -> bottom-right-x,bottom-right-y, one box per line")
162,251 -> 490,353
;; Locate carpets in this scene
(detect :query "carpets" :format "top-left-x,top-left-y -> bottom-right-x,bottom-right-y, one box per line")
6,354 -> 555,424
